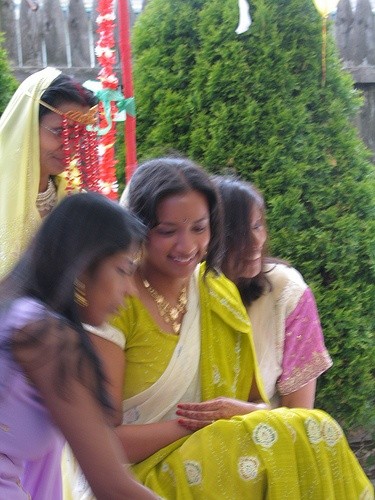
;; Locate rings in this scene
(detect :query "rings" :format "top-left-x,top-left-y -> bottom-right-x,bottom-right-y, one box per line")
211,420 -> 215,423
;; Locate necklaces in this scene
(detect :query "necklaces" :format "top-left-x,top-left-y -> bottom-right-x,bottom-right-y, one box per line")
135,256 -> 188,334
36,178 -> 57,212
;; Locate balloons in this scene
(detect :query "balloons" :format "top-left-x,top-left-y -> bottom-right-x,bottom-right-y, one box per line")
313,0 -> 339,16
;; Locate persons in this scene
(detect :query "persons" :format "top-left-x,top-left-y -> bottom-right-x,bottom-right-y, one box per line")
209,174 -> 333,409
0,192 -> 164,500
61,158 -> 375,500
0,67 -> 99,279
